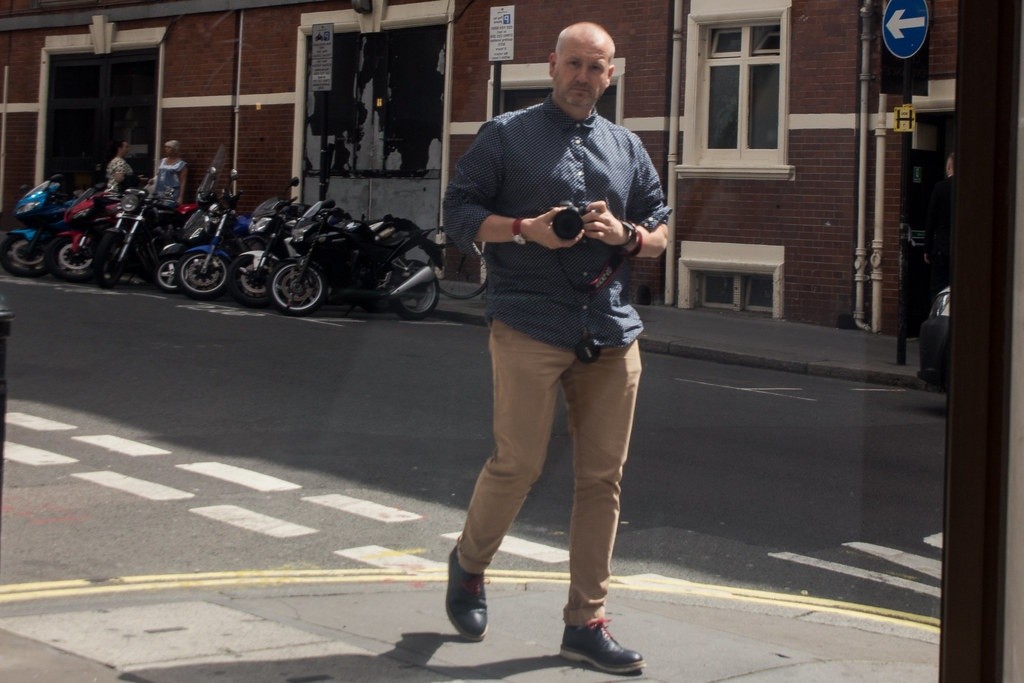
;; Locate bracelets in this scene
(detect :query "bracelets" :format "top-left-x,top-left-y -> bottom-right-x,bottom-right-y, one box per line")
178,196 -> 183,202
612,220 -> 642,256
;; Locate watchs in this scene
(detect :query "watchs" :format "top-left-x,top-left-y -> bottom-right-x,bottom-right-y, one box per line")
512,217 -> 527,244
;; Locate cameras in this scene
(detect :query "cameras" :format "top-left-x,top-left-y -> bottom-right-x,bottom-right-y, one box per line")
549,199 -> 592,240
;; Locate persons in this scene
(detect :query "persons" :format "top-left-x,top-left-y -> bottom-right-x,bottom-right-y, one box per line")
148,140 -> 187,203
923,152 -> 955,302
107,142 -> 147,192
441,20 -> 674,670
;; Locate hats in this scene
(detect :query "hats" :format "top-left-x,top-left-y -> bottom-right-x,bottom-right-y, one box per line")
164,139 -> 180,150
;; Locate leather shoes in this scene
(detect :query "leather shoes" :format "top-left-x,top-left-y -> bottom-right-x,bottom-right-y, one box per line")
445,545 -> 489,640
560,620 -> 646,673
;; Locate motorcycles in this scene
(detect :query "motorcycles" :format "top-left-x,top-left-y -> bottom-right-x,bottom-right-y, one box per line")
0,163 -> 445,322
